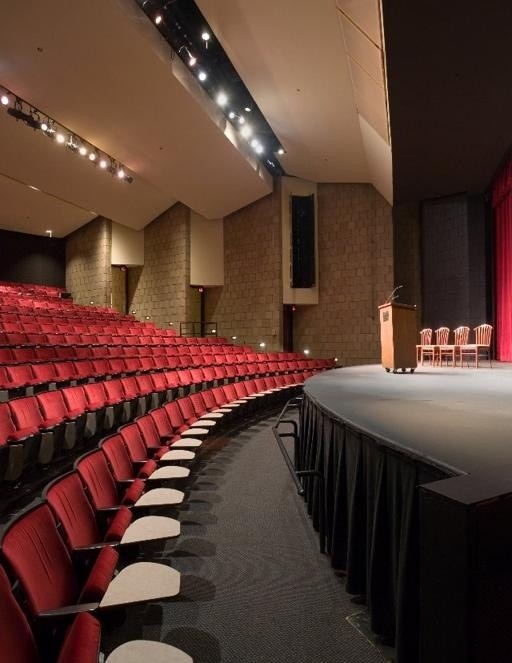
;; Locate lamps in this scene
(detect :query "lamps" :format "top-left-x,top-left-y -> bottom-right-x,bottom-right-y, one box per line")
134,0 -> 287,178
0,85 -> 134,184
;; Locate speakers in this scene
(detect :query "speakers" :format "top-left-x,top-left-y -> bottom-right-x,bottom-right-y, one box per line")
290,193 -> 315,289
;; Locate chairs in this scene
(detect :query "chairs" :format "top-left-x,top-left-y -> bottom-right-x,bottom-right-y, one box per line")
0,280 -> 343,663
415,323 -> 493,369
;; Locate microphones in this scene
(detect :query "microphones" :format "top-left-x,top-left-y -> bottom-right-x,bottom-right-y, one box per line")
385,285 -> 404,303
